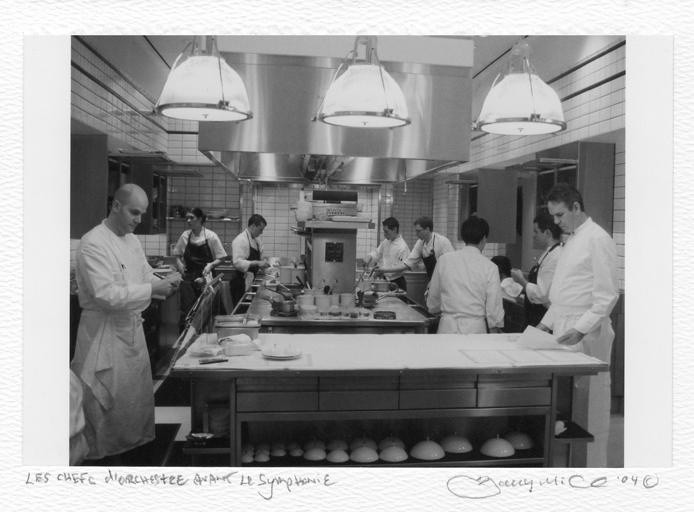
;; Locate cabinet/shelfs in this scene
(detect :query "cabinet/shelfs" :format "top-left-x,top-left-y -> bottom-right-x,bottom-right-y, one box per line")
71,134 -> 167,237
537,142 -> 614,247
140,286 -> 181,370
444,170 -> 517,245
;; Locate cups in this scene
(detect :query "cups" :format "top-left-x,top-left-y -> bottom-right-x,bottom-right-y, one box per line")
296,289 -> 355,310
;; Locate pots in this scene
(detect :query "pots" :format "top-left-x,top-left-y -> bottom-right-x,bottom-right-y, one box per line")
259,287 -> 299,316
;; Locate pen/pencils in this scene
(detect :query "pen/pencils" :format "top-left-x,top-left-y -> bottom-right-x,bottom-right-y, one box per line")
153,273 -> 178,287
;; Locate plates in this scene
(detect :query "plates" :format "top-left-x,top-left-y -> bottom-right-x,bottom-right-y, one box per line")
206,208 -> 228,218
262,347 -> 304,360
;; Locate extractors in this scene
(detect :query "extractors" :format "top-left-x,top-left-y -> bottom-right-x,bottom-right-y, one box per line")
190,47 -> 477,187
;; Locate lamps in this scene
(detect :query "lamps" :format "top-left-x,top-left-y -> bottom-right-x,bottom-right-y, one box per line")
471,36 -> 567,136
150,36 -> 255,125
310,36 -> 413,134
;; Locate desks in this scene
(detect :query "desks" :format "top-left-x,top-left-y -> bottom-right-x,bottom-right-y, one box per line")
171,330 -> 609,466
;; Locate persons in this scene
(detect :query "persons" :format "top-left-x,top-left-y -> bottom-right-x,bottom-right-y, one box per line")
373,216 -> 453,285
362,215 -> 411,295
490,255 -> 524,302
69,183 -> 185,465
422,216 -> 507,334
230,212 -> 269,314
510,210 -> 566,333
534,183 -> 620,468
172,205 -> 228,338
